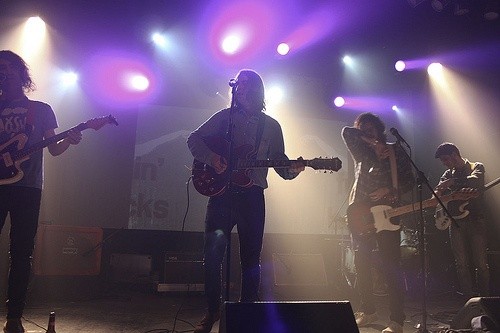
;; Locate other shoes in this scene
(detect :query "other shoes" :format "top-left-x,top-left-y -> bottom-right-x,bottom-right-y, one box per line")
3,318 -> 27,333
194,304 -> 222,333
355,310 -> 380,326
381,321 -> 404,333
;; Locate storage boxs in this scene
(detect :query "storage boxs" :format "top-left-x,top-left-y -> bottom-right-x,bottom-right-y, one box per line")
34,225 -> 103,276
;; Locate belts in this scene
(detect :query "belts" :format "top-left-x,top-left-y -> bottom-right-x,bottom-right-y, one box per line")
208,191 -> 263,202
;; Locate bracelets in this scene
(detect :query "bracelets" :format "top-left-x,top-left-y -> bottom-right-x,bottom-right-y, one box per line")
371,138 -> 379,146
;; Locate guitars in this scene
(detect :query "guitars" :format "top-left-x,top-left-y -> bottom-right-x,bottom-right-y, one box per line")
190,136 -> 332,198
0,113 -> 121,187
345,187 -> 481,236
434,175 -> 500,233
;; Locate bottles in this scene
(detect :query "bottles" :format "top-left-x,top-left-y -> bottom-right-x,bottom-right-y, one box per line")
45,312 -> 56,333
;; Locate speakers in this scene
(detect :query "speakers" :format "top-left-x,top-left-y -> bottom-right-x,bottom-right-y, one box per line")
160,251 -> 222,282
272,252 -> 328,287
450,296 -> 500,331
33,225 -> 103,277
324,239 -> 358,289
219,301 -> 359,333
110,252 -> 151,285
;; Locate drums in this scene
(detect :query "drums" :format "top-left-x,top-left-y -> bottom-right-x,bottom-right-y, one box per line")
397,227 -> 420,257
345,244 -> 361,274
372,248 -> 388,297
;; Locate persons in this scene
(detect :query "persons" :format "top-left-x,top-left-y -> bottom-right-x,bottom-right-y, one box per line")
431,143 -> 490,313
187,70 -> 306,333
0,49 -> 82,333
341,113 -> 416,333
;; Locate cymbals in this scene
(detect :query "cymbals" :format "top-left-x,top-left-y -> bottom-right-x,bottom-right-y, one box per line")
324,239 -> 351,242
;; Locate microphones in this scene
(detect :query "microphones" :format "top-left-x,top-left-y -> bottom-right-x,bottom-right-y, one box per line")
229,79 -> 240,87
390,128 -> 411,150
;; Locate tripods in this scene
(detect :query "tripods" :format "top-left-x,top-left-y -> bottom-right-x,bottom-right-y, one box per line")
328,219 -> 353,289
